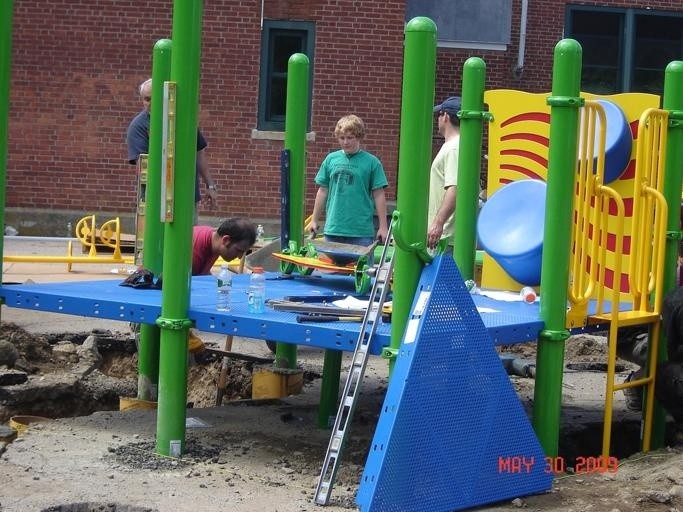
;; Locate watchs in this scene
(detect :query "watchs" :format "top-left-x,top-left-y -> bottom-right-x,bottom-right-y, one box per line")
207,182 -> 217,190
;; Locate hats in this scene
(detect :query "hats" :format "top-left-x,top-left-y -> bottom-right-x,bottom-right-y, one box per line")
433,96 -> 461,112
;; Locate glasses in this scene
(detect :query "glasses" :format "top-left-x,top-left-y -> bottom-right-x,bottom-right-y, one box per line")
435,110 -> 448,121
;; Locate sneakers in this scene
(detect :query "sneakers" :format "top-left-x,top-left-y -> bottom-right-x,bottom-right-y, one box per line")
623,371 -> 642,410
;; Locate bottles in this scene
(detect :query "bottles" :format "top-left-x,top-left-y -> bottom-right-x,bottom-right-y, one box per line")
246,266 -> 266,314
257,224 -> 265,245
66,222 -> 73,237
519,286 -> 537,306
215,262 -> 234,312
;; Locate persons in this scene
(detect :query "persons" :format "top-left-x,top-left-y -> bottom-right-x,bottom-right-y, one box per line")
620,241 -> 683,427
126,77 -> 218,227
307,115 -> 389,275
131,218 -> 256,367
425,95 -> 463,267
